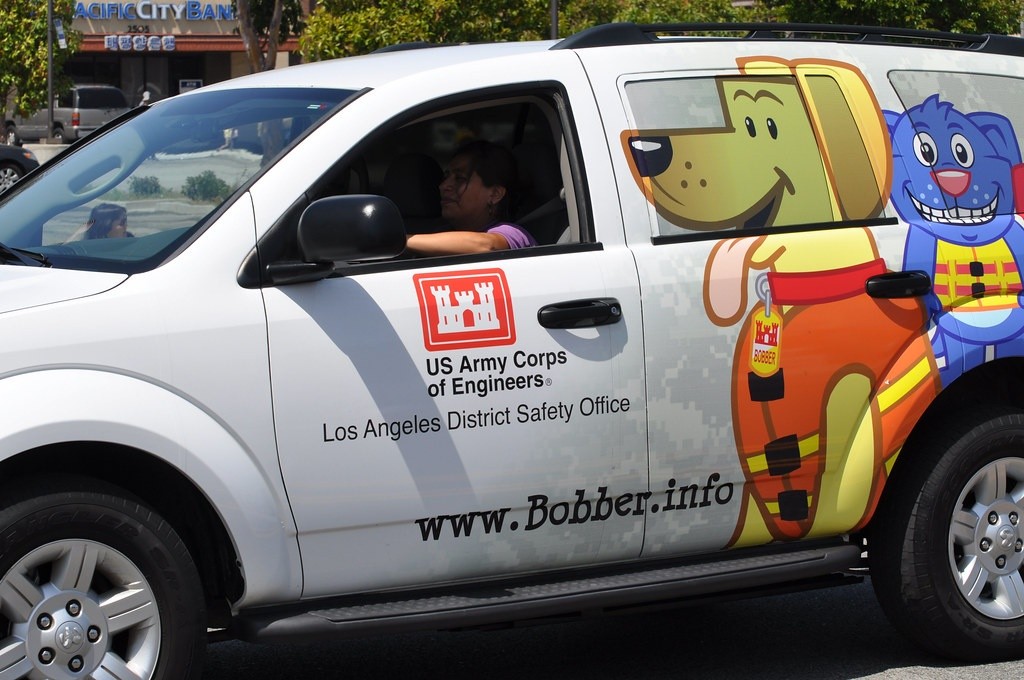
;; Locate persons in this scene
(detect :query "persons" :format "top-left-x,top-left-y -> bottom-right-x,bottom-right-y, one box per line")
138,91 -> 159,161
407,141 -> 537,257
61,202 -> 134,245
216,126 -> 240,152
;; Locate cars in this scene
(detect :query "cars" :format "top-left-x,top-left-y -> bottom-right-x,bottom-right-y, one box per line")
0,145 -> 40,196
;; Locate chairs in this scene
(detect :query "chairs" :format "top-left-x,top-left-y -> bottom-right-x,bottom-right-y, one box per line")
508,145 -> 569,246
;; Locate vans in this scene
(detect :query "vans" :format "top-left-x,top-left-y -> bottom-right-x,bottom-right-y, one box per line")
4,84 -> 131,147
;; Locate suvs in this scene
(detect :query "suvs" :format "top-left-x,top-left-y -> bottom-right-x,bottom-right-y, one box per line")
0,22 -> 1024,680
